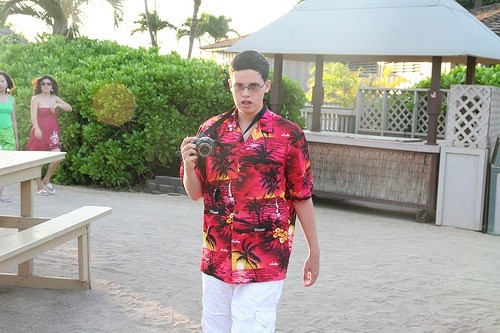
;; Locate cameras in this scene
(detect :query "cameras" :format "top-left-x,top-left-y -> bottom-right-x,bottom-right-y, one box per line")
189,137 -> 215,157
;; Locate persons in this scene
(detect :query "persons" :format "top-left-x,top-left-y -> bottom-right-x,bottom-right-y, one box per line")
180,49 -> 319,333
0,71 -> 18,202
27,74 -> 72,196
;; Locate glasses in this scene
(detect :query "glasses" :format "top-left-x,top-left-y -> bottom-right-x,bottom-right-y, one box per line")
232,81 -> 264,91
40,81 -> 52,85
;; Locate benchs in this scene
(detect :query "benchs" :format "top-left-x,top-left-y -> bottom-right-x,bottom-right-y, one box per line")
0,206 -> 114,290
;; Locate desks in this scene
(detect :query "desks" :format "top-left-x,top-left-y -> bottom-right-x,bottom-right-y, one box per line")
0,149 -> 67,285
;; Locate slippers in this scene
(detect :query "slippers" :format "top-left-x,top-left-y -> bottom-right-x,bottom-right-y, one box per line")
35,189 -> 49,195
42,181 -> 55,192
